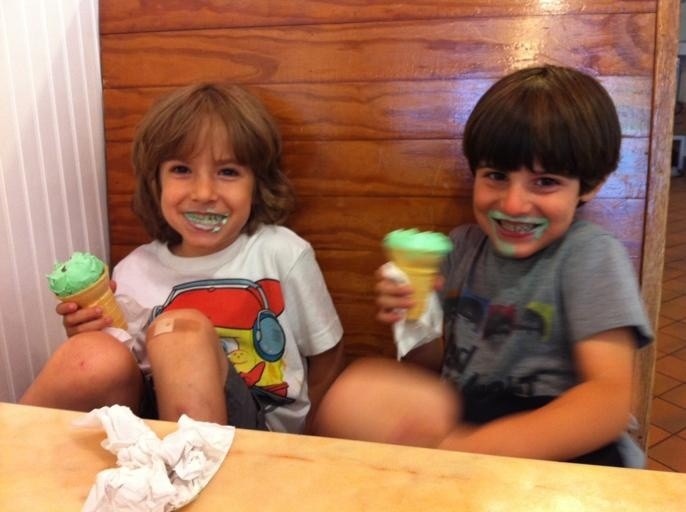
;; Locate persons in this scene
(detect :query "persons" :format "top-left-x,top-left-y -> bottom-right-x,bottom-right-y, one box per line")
309,65 -> 655,477
13,79 -> 350,439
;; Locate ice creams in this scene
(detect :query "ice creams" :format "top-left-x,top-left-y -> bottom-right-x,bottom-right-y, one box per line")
46,250 -> 129,334
380,228 -> 453,323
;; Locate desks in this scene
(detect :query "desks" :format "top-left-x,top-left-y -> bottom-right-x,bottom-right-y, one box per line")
0,402 -> 686,512
